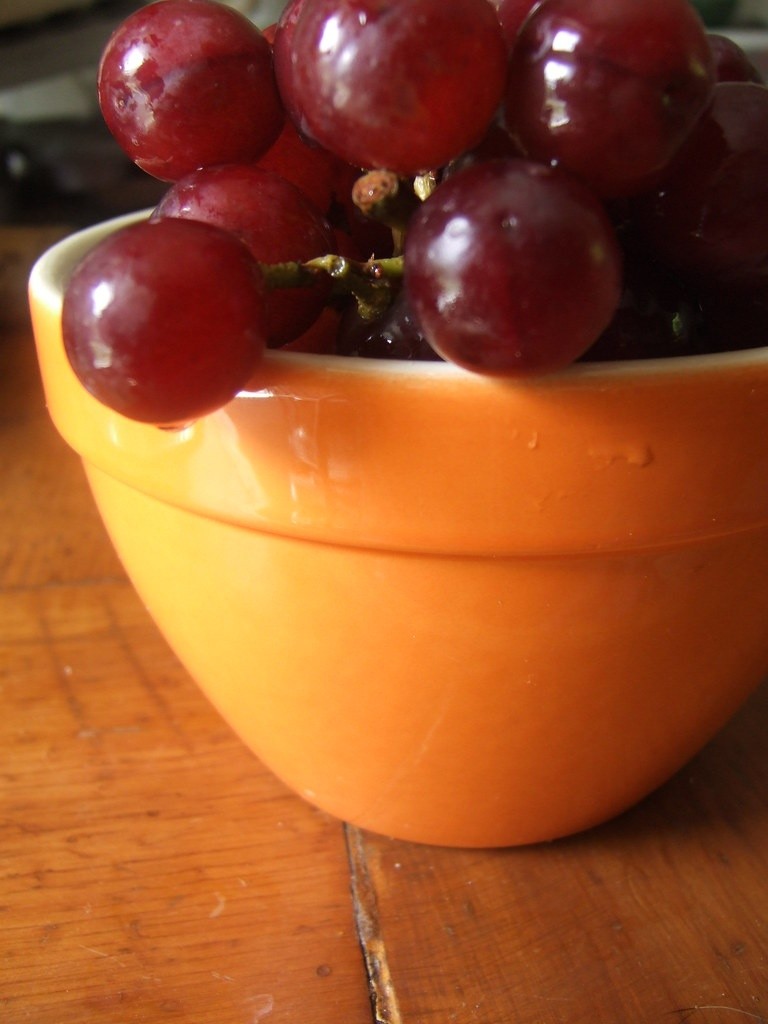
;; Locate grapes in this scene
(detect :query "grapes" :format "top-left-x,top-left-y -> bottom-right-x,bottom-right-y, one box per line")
61,0 -> 768,424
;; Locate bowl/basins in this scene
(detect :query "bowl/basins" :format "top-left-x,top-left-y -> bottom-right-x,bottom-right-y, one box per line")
28,205 -> 768,849
